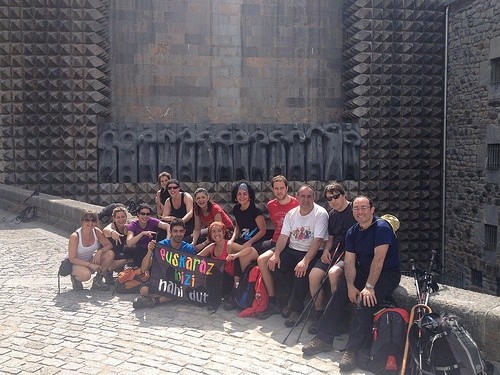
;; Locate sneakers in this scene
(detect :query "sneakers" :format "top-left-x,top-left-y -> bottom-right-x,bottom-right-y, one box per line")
339,352 -> 356,370
255,304 -> 282,319
302,338 -> 333,355
285,311 -> 304,327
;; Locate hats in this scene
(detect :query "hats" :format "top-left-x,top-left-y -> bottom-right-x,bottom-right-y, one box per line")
380,214 -> 400,238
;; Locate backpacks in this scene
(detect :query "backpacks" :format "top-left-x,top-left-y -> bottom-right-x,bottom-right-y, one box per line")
357,308 -> 410,375
409,312 -> 486,375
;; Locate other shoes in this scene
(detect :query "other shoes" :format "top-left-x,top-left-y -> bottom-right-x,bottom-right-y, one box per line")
308,323 -> 320,333
71,276 -> 83,291
224,299 -> 237,309
93,278 -> 110,291
132,297 -> 153,309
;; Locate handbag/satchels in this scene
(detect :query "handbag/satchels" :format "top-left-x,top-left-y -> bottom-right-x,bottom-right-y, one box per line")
59,259 -> 72,276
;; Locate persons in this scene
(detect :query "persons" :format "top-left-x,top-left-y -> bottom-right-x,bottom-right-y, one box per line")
238,176 -> 300,322
266,185 -> 330,327
126,171 -> 265,316
308,183 -> 358,334
102,206 -> 136,285
65,210 -> 116,291
302,196 -> 401,370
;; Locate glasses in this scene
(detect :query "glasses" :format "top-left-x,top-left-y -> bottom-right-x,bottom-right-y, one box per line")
327,193 -> 341,201
352,206 -> 370,211
166,186 -> 179,190
84,218 -> 97,223
138,212 -> 151,216
